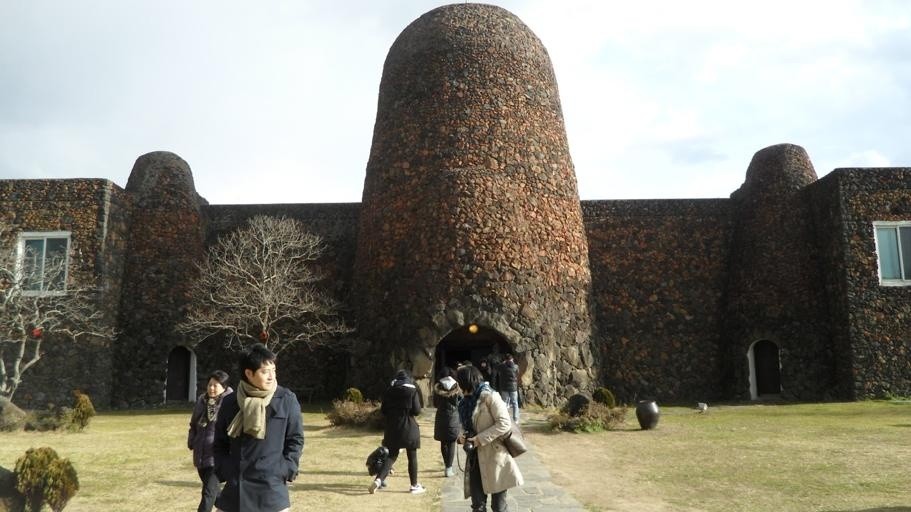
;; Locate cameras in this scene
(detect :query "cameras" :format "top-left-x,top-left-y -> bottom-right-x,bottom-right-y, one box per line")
462,431 -> 478,450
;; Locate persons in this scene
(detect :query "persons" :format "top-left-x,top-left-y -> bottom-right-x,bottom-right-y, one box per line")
432,366 -> 456,477
367,369 -> 427,496
213,342 -> 303,511
452,363 -> 521,512
457,351 -> 520,425
186,366 -> 233,512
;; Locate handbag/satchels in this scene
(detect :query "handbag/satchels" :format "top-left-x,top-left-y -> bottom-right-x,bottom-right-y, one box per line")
504,417 -> 527,457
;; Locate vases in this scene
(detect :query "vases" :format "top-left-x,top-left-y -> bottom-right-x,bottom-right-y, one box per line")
635,400 -> 659,430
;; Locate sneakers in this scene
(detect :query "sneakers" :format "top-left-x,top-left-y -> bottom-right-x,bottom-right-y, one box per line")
410,484 -> 425,494
445,467 -> 453,476
368,479 -> 381,493
